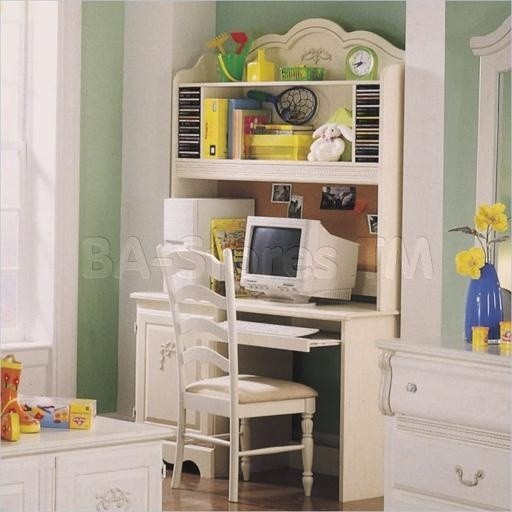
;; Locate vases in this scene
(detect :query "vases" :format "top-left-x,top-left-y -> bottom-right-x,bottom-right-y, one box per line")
464,263 -> 503,344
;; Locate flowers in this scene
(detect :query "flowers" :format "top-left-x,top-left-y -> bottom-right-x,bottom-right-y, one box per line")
447,201 -> 512,281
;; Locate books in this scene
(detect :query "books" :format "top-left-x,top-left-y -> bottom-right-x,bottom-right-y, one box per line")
209,217 -> 247,295
256,122 -> 312,131
236,108 -> 238,160
239,109 -> 272,161
258,128 -> 314,135
228,98 -> 262,159
232,109 -> 235,160
215,227 -> 254,299
203,97 -> 228,159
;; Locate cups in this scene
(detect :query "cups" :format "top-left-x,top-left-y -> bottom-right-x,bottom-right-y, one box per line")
501,321 -> 512,347
472,325 -> 489,347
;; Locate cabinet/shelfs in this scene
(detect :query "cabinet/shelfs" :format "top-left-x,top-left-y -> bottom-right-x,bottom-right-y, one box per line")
163,17 -> 406,314
376,335 -> 512,512
0,416 -> 173,512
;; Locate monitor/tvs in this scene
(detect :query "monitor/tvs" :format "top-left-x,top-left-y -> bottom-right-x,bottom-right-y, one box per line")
240,216 -> 360,306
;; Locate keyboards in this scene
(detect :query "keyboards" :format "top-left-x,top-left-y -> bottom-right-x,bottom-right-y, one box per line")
220,319 -> 320,338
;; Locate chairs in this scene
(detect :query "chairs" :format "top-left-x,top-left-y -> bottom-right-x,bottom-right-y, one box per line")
155,244 -> 319,502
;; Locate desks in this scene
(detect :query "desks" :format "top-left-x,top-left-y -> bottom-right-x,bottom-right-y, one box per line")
127,290 -> 401,502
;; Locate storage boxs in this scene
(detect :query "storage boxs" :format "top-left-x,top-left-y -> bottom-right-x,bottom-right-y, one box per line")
246,134 -> 315,162
